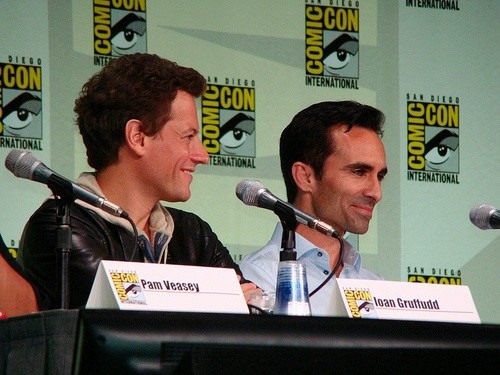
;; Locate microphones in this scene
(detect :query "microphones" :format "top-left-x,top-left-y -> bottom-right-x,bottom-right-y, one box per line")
235,179 -> 340,238
469,203 -> 500,230
4,150 -> 129,219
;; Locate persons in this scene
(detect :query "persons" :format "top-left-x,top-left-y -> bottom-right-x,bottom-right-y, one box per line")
15,53 -> 267,310
236,100 -> 388,317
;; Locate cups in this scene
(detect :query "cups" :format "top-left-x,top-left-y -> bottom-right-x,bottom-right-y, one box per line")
272,261 -> 312,316
249,292 -> 275,315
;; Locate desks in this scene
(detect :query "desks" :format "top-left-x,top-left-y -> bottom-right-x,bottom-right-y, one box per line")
0,308 -> 500,375
